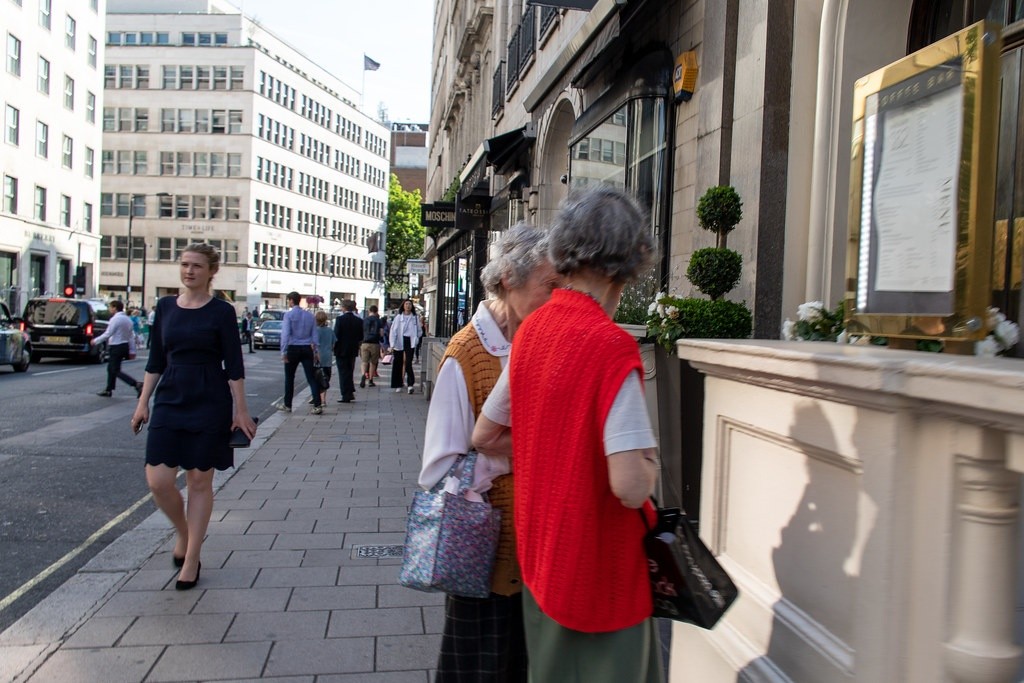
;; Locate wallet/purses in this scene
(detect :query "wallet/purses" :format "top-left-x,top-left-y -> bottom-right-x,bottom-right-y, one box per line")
229,417 -> 258,448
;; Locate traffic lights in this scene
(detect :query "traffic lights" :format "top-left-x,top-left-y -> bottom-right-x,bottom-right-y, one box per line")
64,284 -> 74,298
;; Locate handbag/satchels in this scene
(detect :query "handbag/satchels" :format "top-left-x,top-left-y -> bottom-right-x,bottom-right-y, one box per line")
381,354 -> 394,365
397,451 -> 503,598
638,457 -> 738,630
313,360 -> 330,393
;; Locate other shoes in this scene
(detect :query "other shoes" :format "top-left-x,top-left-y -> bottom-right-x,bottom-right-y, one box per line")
275,403 -> 292,412
360,375 -> 366,388
408,386 -> 415,393
414,359 -> 420,364
365,372 -> 369,379
337,398 -> 350,403
368,382 -> 375,386
249,351 -> 256,353
309,398 -> 314,404
396,388 -> 402,392
321,404 -> 326,407
373,371 -> 378,377
310,406 -> 322,414
351,395 -> 355,400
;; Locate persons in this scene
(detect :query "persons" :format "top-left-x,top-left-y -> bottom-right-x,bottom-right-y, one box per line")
275,292 -> 322,414
418,223 -> 567,683
126,307 -> 158,350
90,300 -> 144,399
472,184 -> 665,683
240,306 -> 260,354
130,244 -> 256,590
314,299 -> 426,403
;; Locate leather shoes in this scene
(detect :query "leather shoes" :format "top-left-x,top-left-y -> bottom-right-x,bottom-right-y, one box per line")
96,390 -> 112,397
135,382 -> 143,398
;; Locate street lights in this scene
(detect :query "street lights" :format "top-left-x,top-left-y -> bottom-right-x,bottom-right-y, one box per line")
124,192 -> 172,312
313,234 -> 340,309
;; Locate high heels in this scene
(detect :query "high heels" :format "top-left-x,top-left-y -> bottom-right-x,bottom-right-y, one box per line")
173,555 -> 185,565
175,561 -> 201,589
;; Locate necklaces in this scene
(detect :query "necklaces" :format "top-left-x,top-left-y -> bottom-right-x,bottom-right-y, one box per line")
562,284 -> 603,307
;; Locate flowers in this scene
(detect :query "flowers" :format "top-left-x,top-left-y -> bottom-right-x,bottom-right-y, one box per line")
786,302 -> 1024,359
644,289 -> 689,355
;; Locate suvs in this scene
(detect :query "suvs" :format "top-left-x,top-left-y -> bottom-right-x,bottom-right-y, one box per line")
22,296 -> 115,364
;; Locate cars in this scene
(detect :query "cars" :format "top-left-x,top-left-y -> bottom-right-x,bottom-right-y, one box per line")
0,298 -> 31,373
255,307 -> 342,325
253,320 -> 283,348
